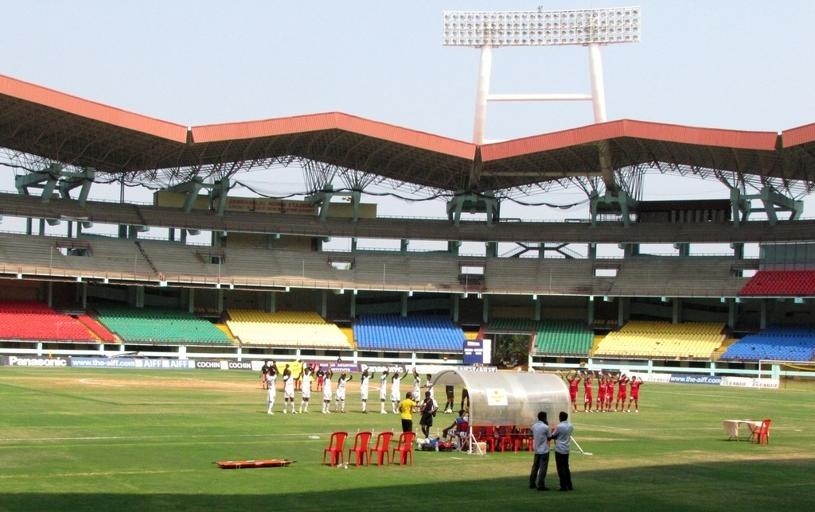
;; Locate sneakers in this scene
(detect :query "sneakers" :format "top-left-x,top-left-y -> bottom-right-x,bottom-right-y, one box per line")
267,408 -> 400,415
575,410 -> 639,413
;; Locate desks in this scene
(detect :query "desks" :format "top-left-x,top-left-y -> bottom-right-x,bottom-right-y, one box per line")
724,419 -> 770,442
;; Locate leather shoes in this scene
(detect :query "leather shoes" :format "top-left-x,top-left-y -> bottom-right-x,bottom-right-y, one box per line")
528,485 -> 549,491
556,487 -> 573,491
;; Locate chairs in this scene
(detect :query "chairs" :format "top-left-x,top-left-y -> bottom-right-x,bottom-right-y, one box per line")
321,430 -> 417,470
450,425 -> 533,453
749,417 -> 772,447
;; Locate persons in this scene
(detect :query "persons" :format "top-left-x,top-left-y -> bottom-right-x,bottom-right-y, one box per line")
418,391 -> 437,437
558,370 -> 644,412
526,410 -> 577,492
444,410 -> 468,447
262,359 -> 470,414
398,392 -> 420,434
